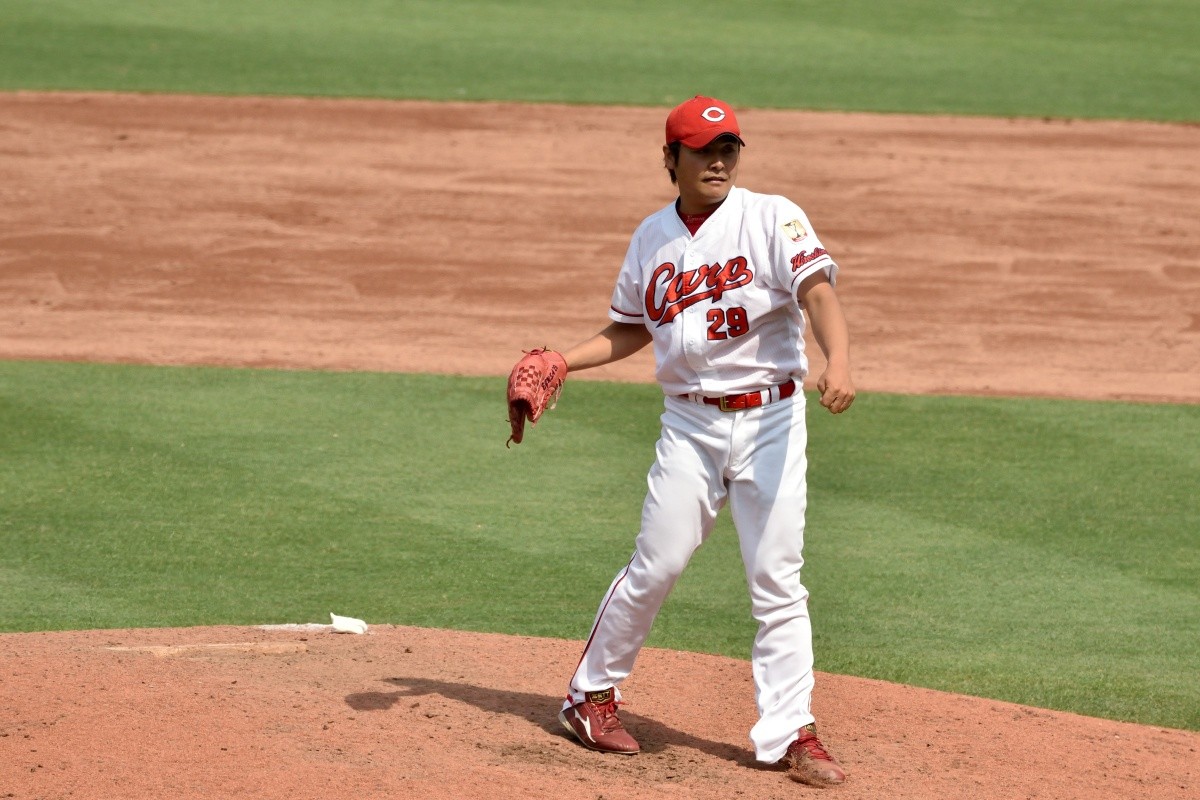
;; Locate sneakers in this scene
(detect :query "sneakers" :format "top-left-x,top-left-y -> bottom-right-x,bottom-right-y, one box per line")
777,724 -> 845,785
558,686 -> 640,754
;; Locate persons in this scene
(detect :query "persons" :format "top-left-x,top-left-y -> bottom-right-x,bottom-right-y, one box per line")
510,97 -> 855,786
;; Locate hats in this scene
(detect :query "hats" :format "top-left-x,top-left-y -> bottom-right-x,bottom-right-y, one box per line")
665,95 -> 746,150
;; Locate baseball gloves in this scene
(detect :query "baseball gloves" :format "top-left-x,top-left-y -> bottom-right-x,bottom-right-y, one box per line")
503,345 -> 568,449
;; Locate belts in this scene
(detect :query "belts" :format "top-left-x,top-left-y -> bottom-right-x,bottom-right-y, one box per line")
680,379 -> 796,412
